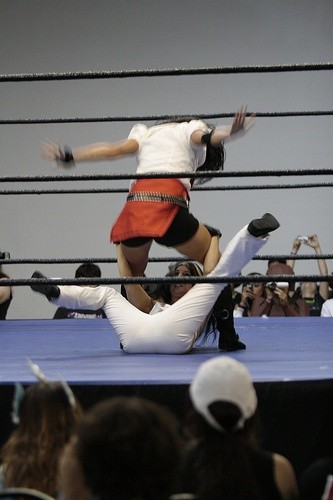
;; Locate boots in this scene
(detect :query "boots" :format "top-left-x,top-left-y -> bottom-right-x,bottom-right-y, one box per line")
213,285 -> 247,351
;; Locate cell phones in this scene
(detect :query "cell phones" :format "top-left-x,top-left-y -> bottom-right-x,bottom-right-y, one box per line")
246,284 -> 253,295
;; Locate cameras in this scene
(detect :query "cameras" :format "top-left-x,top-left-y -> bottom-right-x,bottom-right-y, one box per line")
266,281 -> 289,290
298,237 -> 309,242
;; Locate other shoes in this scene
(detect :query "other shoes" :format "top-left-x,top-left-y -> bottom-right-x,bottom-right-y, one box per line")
247,213 -> 280,237
30,270 -> 61,300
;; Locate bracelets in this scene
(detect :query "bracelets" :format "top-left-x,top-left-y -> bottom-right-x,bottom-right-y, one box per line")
291,252 -> 297,254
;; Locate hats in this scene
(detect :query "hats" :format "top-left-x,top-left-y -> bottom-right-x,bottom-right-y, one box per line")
190,357 -> 257,433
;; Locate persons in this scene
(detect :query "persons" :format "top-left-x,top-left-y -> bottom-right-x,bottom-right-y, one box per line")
0,352 -> 333,500
39,103 -> 258,351
29,213 -> 280,355
228,232 -> 333,318
51,262 -> 108,320
0,251 -> 14,320
